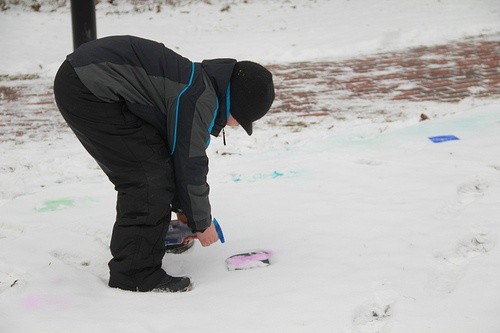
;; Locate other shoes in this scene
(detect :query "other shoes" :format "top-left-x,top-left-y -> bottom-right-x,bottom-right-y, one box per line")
166,239 -> 194,254
152,274 -> 191,292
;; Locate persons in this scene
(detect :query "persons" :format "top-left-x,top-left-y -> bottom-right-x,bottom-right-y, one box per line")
51,35 -> 276,293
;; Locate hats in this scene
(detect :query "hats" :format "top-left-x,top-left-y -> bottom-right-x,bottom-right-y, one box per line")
228,60 -> 275,137
